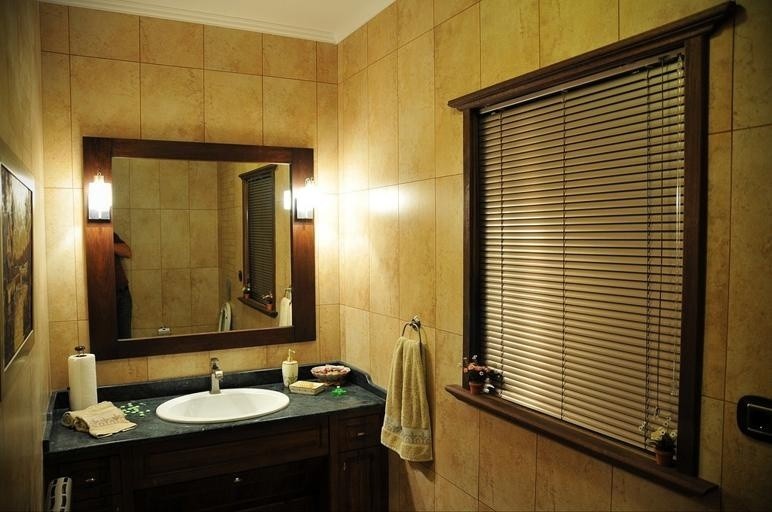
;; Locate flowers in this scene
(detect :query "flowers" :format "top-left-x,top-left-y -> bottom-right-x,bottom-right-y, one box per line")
639,417 -> 677,450
459,355 -> 502,383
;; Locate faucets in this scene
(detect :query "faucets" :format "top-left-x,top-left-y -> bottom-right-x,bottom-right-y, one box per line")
209,355 -> 223,392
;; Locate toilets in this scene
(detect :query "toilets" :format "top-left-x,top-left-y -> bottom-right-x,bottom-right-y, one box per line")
216,302 -> 233,332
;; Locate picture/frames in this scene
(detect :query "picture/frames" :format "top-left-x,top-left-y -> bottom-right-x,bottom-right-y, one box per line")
1,139 -> 36,396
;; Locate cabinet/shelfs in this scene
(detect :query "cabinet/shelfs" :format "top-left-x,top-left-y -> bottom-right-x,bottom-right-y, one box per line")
44,408 -> 390,512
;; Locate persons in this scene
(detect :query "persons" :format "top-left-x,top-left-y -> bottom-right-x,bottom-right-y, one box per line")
114,232 -> 132,339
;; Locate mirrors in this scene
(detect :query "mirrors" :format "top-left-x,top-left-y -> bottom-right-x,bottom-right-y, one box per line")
83,136 -> 316,363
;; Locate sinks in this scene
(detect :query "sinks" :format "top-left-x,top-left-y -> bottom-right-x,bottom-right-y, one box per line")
156,387 -> 289,424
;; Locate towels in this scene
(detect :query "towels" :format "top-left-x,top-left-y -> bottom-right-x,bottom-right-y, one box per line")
75,407 -> 138,438
381,338 -> 432,462
61,400 -> 115,426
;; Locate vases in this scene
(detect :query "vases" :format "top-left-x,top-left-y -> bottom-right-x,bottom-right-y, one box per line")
469,382 -> 480,394
655,449 -> 672,465
